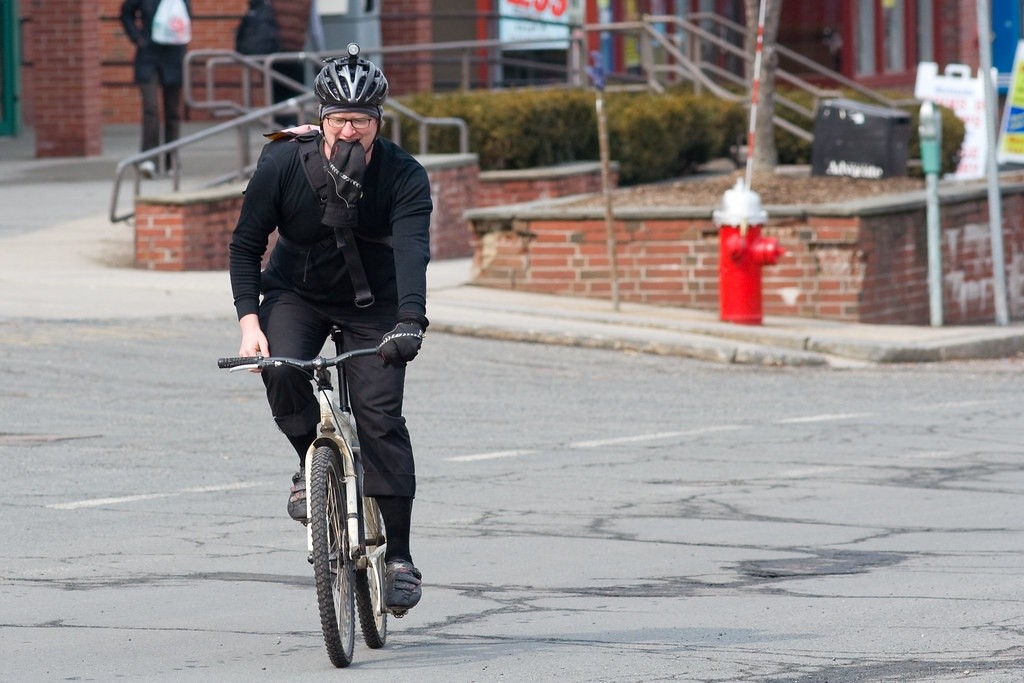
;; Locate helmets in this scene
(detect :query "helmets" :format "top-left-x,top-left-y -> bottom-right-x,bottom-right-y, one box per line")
314,41 -> 389,118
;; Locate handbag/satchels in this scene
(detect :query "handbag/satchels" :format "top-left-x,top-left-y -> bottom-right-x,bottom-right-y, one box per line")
236,0 -> 279,55
151,0 -> 192,45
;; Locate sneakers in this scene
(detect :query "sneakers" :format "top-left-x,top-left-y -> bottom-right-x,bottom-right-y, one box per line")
384,556 -> 422,608
287,465 -> 307,519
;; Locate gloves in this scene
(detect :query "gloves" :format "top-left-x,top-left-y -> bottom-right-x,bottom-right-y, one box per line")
320,137 -> 367,224
378,320 -> 422,368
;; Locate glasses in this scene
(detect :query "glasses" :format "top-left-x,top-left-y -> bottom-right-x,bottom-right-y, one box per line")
325,115 -> 373,129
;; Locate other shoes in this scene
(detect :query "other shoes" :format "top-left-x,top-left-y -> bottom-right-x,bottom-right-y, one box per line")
140,160 -> 158,178
165,164 -> 175,177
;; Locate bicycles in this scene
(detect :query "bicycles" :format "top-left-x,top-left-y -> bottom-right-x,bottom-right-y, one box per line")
217,347 -> 409,668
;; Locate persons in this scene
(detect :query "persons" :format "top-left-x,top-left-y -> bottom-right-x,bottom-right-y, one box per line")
119,0 -> 192,180
227,56 -> 433,615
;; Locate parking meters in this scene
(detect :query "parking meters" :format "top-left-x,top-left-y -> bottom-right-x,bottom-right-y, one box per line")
920,99 -> 948,329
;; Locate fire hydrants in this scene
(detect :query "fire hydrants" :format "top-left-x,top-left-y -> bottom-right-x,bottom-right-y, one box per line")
712,179 -> 785,328
